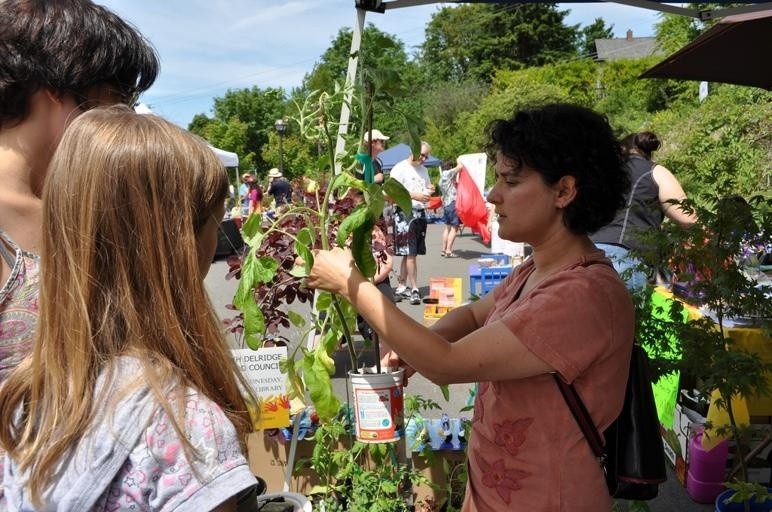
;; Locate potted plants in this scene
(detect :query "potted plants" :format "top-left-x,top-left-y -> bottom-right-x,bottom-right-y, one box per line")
594,104 -> 771,511
225,68 -> 425,448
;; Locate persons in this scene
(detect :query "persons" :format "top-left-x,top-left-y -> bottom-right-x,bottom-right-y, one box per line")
248,169 -> 262,215
588,130 -> 700,305
267,166 -> 294,210
306,97 -> 635,511
239,172 -> 250,214
0,98 -> 264,512
0,1 -> 163,512
353,122 -> 394,286
388,135 -> 437,306
438,152 -> 466,258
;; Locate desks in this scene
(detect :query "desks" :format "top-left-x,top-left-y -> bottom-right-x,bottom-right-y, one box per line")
469,264 -> 514,303
638,277 -> 770,471
213,214 -> 251,263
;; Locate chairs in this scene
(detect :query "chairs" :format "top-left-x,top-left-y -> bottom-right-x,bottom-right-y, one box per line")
480,255 -> 509,266
481,267 -> 511,301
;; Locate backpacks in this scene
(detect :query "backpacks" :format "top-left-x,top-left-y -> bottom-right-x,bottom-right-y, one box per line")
550,257 -> 671,502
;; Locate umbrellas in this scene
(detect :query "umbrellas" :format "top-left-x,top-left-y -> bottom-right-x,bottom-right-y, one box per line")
634,9 -> 772,95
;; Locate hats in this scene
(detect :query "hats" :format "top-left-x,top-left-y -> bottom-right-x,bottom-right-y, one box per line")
267,167 -> 283,178
363,127 -> 390,143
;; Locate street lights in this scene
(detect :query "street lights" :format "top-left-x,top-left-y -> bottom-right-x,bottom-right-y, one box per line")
272,117 -> 287,177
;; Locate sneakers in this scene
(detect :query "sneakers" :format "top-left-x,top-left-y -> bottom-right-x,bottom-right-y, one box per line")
409,287 -> 422,306
440,250 -> 460,259
393,286 -> 413,299
